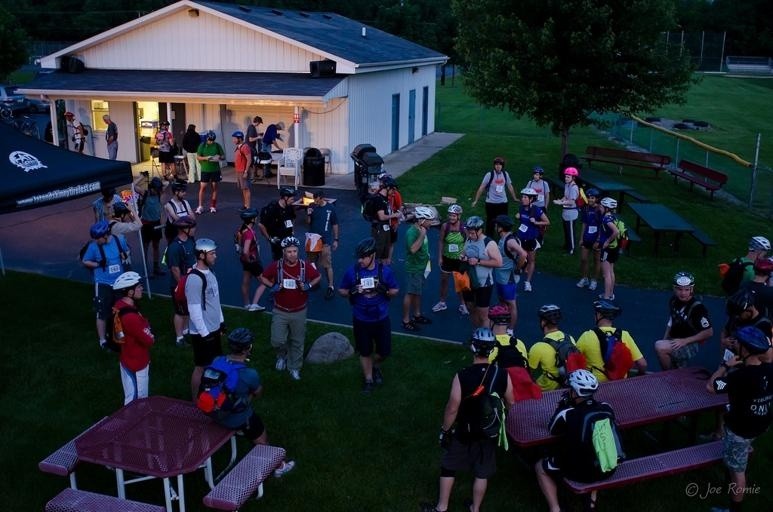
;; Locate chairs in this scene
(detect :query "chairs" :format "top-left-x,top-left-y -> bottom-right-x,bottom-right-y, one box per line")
275,147 -> 333,192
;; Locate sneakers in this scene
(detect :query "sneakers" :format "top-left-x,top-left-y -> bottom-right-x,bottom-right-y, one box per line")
242,283 -> 447,394
418,502 -> 446,511
463,497 -> 477,512
513,268 -> 615,299
275,459 -> 295,478
174,336 -> 192,351
99,338 -> 116,354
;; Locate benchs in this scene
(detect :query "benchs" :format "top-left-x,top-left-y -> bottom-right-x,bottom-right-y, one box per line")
34,415 -> 286,509
611,212 -> 718,257
543,174 -> 565,193
578,144 -> 729,202
554,437 -> 729,511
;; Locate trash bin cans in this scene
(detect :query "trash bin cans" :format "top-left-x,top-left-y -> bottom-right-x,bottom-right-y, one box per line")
304,148 -> 325,186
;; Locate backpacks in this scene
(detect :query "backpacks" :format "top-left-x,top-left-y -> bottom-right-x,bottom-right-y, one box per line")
466,300 -> 622,399
196,356 -> 245,417
602,214 -> 629,251
568,401 -> 618,482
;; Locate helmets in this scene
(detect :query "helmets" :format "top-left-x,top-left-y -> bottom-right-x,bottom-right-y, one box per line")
415,204 -> 513,232
227,328 -> 253,353
232,116 -> 396,257
672,237 -> 773,357
89,118 -> 220,298
495,157 -> 619,210
64,112 -> 74,117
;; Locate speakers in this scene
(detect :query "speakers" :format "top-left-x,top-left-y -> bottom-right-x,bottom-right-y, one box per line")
310,61 -> 336,77
60,56 -> 84,73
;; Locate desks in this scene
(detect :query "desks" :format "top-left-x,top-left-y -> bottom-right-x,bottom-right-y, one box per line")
489,361 -> 727,447
73,391 -> 237,511
577,170 -> 636,203
627,199 -> 694,253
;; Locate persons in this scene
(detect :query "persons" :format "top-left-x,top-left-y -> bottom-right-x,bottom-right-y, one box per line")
0,106 -> 12,124
63,111 -> 85,154
77,113 -> 772,512
